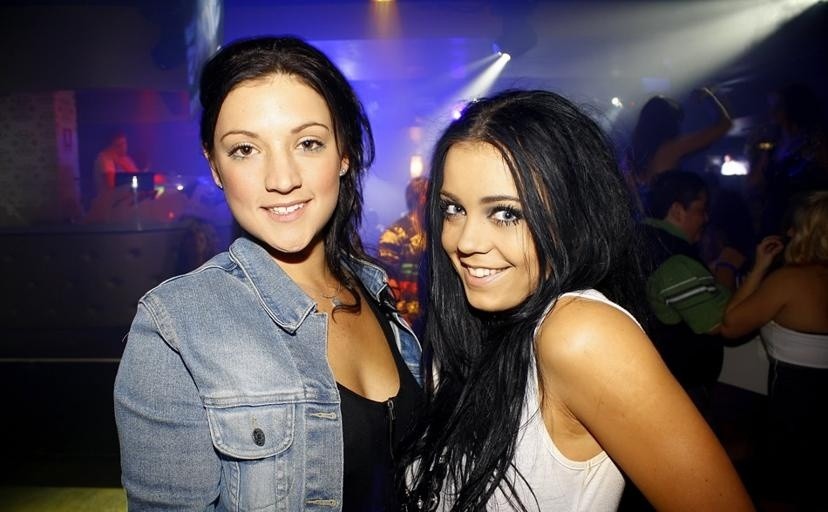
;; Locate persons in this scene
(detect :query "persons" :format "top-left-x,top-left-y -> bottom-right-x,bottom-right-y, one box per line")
398,88 -> 757,511
111,33 -> 422,512
374,161 -> 827,478
182,229 -> 208,270
94,129 -> 137,190
629,86 -> 734,187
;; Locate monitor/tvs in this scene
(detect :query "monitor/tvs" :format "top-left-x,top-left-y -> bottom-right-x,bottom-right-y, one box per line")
115,171 -> 154,192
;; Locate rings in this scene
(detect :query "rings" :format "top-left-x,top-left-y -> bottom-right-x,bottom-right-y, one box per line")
320,291 -> 345,308
